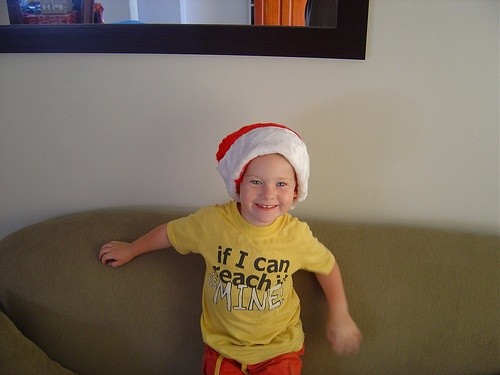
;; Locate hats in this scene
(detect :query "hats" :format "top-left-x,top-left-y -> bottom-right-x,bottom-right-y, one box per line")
216,123 -> 309,212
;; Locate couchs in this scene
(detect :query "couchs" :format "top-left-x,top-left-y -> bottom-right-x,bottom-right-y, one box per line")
0,204 -> 500,375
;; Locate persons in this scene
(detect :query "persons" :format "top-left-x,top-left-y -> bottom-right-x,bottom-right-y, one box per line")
97,121 -> 365,375
